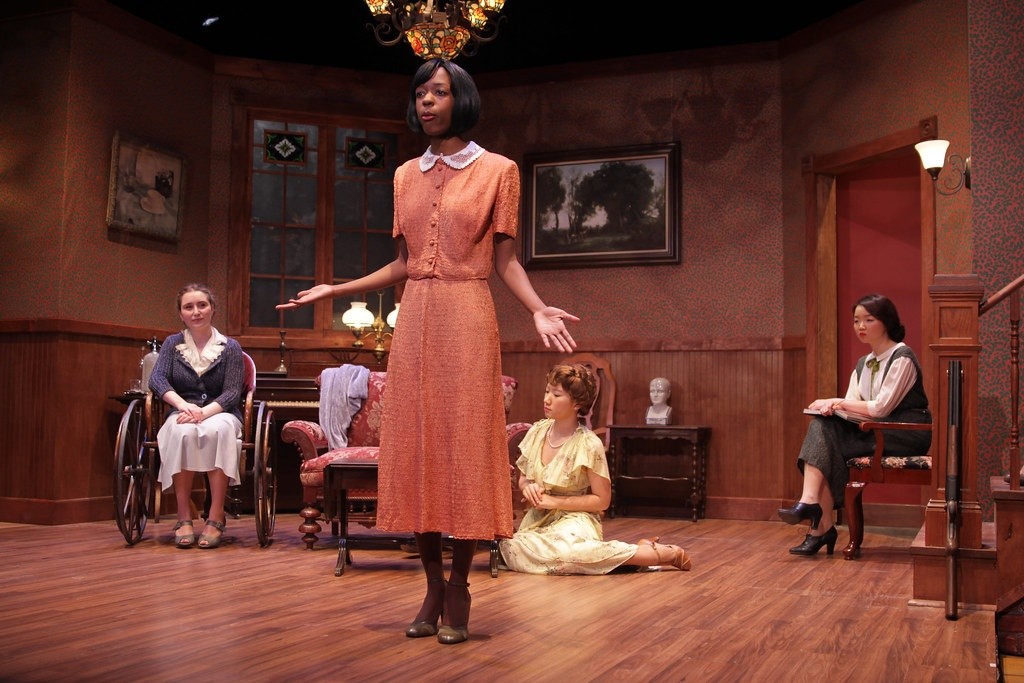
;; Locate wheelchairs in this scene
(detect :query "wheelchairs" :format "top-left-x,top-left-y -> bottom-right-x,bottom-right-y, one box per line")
112,350 -> 277,547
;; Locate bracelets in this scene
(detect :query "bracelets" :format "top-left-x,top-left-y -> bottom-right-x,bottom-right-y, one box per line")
836,399 -> 846,405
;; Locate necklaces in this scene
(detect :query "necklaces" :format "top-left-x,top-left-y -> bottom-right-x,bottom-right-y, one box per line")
548,426 -> 579,448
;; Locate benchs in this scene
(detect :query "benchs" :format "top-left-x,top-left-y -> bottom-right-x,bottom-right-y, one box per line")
281,370 -> 533,549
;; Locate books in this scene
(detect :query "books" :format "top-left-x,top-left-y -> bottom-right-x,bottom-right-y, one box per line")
803,409 -> 872,423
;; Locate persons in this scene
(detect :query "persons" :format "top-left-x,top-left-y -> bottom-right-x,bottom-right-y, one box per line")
645,378 -> 672,425
499,364 -> 690,576
776,295 -> 931,555
275,60 -> 582,642
148,283 -> 245,548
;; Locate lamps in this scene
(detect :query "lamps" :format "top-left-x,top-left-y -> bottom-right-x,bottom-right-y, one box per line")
915,140 -> 971,195
363,0 -> 509,62
341,293 -> 401,348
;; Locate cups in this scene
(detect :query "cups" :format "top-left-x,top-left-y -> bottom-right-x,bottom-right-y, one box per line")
130,379 -> 141,392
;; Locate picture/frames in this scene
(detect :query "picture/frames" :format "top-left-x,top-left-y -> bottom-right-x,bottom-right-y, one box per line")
522,138 -> 682,271
105,129 -> 187,242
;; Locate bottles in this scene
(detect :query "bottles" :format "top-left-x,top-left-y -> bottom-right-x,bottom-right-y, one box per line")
135,336 -> 162,392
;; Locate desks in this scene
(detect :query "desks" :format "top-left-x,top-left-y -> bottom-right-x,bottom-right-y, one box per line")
110,393 -> 200,523
604,424 -> 711,522
323,457 -> 501,577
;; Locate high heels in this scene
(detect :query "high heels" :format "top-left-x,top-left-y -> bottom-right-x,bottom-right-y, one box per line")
789,525 -> 838,556
405,578 -> 471,644
637,536 -> 691,571
777,503 -> 823,530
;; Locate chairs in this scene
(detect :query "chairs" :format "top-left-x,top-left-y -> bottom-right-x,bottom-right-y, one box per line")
560,352 -> 616,521
843,420 -> 932,559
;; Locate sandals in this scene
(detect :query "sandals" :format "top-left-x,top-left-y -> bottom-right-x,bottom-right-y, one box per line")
172,517 -> 227,549
